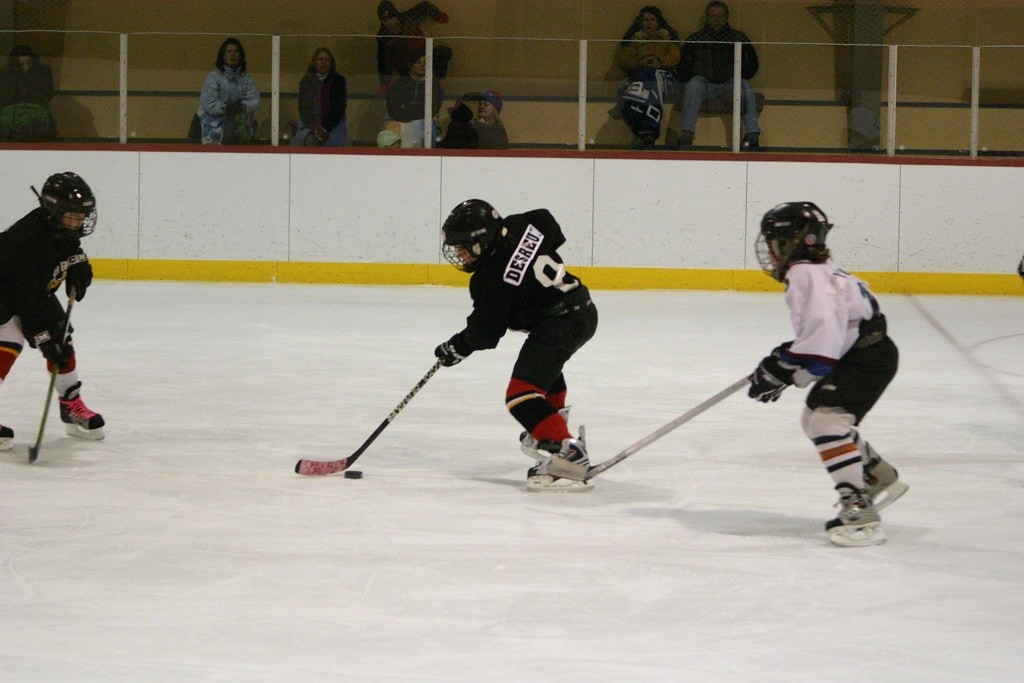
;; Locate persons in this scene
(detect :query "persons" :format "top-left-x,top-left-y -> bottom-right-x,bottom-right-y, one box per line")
607,0 -> 761,151
0,170 -> 110,451
434,198 -> 598,496
291,44 -> 349,147
376,0 -> 508,149
191,37 -> 261,147
0,43 -> 59,144
749,202 -> 909,551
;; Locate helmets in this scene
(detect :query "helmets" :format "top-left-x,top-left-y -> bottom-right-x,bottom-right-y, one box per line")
30,171 -> 97,241
754,201 -> 834,282
440,198 -> 504,274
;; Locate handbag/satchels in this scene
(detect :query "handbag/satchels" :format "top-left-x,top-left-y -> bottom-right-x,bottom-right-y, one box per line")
0,99 -> 58,142
633,65 -> 656,81
187,113 -> 202,139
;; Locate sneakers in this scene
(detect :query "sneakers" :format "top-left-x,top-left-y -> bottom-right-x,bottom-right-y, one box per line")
527,423 -> 591,479
862,441 -> 898,499
59,381 -> 105,430
825,482 -> 881,531
519,404 -> 571,450
0,425 -> 13,438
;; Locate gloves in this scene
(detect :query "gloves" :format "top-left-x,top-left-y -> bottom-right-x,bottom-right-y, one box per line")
226,102 -> 242,114
379,84 -> 391,95
747,355 -> 795,403
65,263 -> 91,301
39,337 -> 74,368
312,125 -> 329,145
438,10 -> 449,23
434,333 -> 473,367
771,341 -> 792,361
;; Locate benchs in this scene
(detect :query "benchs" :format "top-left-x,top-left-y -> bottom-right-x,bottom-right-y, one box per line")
62,88 -> 1024,151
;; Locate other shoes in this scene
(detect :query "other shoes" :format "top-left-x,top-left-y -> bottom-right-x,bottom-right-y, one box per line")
630,134 -> 656,150
678,130 -> 694,146
607,104 -> 624,120
742,133 -> 759,149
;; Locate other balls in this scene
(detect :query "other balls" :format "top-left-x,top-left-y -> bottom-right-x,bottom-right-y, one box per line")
344,471 -> 363,478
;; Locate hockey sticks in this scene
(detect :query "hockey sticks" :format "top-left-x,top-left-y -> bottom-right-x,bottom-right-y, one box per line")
19,285 -> 80,464
294,355 -> 454,475
543,375 -> 760,483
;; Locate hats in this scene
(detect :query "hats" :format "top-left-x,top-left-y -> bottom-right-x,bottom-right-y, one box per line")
377,129 -> 400,149
378,0 -> 399,21
448,103 -> 473,123
479,89 -> 503,114
